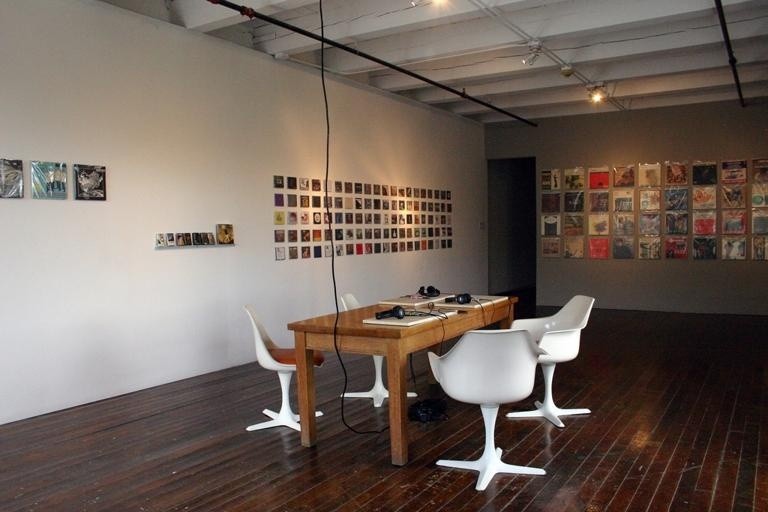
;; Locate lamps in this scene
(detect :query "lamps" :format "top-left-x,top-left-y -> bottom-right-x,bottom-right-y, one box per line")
583,81 -> 605,91
521,40 -> 543,66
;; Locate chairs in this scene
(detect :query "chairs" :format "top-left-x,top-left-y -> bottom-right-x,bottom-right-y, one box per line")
340,293 -> 418,410
426,295 -> 595,491
246,305 -> 326,432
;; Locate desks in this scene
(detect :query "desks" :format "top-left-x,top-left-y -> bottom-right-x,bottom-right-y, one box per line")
287,296 -> 519,466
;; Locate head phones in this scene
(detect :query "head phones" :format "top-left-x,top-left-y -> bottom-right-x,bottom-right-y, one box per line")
375,305 -> 405,320
445,293 -> 472,304
418,285 -> 441,297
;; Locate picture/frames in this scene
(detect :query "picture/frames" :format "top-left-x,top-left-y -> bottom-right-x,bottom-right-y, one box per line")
73,163 -> 106,200
